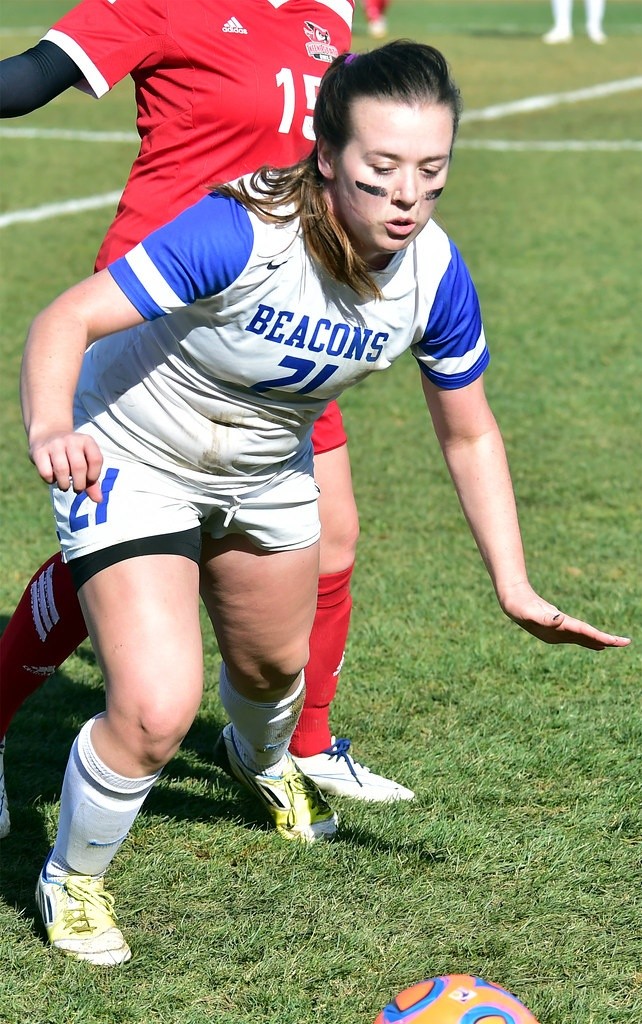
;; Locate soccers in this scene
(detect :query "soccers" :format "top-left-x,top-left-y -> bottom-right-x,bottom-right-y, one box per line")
373,974 -> 541,1024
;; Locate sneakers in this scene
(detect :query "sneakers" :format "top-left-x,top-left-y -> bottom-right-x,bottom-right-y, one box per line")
287,735 -> 415,802
0,735 -> 11,839
213,721 -> 339,843
35,843 -> 132,965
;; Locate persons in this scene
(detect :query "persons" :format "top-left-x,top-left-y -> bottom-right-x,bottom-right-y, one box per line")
361,0 -> 609,44
19,38 -> 632,965
1,1 -> 413,845
